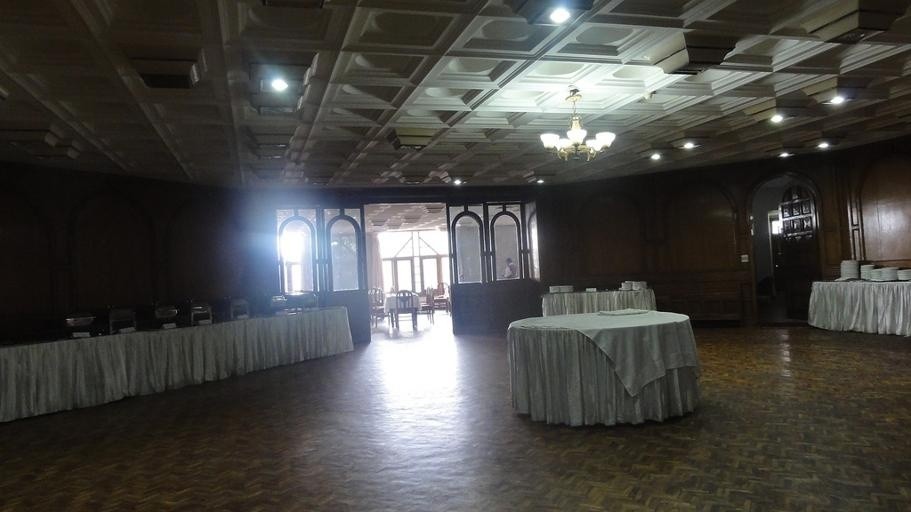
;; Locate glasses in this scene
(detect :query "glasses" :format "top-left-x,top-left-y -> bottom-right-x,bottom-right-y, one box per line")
540,90 -> 617,163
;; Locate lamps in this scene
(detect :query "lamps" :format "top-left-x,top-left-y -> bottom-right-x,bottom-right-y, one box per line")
62,295 -> 288,339
368,282 -> 450,328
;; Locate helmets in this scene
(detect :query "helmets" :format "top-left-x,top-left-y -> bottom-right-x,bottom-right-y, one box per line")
618,281 -> 641,290
548,285 -> 574,293
840,259 -> 911,281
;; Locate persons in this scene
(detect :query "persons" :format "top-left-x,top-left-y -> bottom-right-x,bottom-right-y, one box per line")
504,258 -> 517,278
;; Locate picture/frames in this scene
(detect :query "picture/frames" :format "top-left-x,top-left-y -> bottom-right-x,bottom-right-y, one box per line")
808,281 -> 911,337
0,305 -> 354,423
